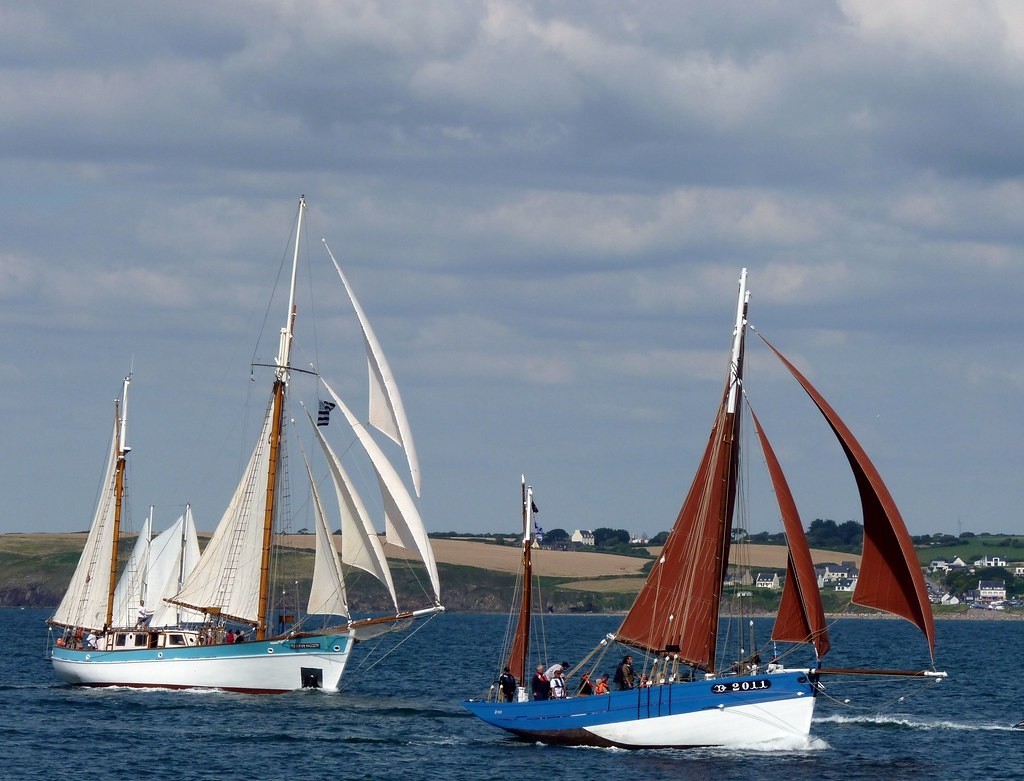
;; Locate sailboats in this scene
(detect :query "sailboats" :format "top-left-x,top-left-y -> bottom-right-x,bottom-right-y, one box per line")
454,265 -> 952,754
39,190 -> 451,695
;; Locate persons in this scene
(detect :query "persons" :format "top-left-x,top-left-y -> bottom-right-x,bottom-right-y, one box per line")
731,661 -> 741,675
770,655 -> 778,664
135,599 -> 154,632
226,629 -> 244,645
747,661 -> 757,676
499,656 -> 648,703
65,624 -> 107,650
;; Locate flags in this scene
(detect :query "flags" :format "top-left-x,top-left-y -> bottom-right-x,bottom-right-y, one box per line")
317,398 -> 336,427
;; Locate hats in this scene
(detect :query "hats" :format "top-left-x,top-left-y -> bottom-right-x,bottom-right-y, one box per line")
504,667 -> 510,672
562,662 -> 570,667
603,673 -> 610,678
734,661 -> 738,664
583,668 -> 592,676
554,670 -> 561,673
